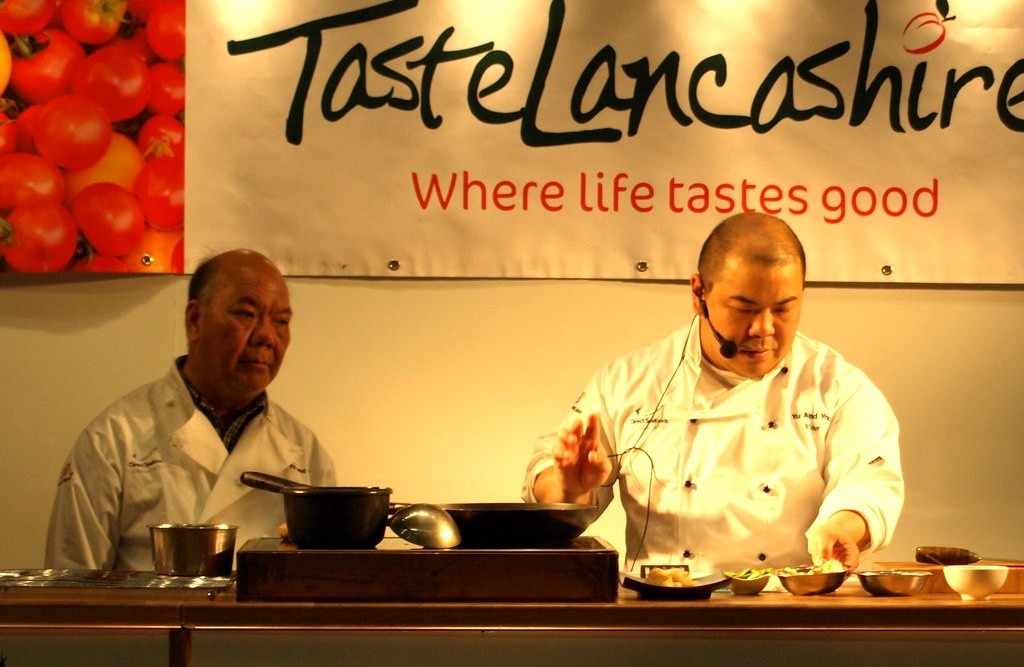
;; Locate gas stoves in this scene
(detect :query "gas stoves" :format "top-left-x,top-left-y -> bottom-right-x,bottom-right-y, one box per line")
237,537 -> 618,602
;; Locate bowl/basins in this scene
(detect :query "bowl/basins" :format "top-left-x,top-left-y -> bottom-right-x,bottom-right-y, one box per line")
943,565 -> 1008,601
723,572 -> 771,595
856,570 -> 933,596
778,569 -> 847,595
145,523 -> 238,577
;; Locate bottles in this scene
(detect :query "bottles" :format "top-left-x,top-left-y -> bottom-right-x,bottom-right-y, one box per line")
916,547 -> 981,565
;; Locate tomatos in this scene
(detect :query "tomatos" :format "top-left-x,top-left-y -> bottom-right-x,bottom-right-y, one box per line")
0,0 -> 186,275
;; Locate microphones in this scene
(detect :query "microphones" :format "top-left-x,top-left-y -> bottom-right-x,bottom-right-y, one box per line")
699,300 -> 737,358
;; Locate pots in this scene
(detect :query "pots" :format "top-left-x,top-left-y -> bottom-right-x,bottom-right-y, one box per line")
240,472 -> 393,550
388,502 -> 597,548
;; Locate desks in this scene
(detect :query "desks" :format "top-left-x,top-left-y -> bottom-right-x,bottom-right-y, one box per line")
0,566 -> 1024,667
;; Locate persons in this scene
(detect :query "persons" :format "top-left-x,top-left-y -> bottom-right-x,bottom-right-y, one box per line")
521,213 -> 905,577
46,248 -> 339,573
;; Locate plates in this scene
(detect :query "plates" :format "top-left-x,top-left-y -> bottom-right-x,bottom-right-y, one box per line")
619,570 -> 732,599
